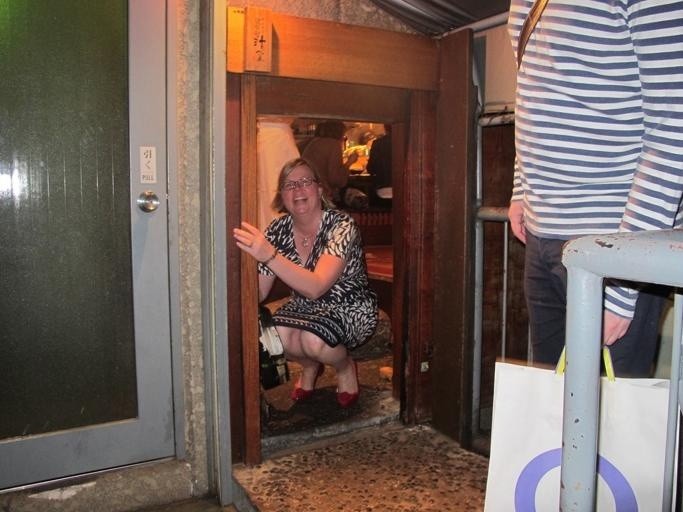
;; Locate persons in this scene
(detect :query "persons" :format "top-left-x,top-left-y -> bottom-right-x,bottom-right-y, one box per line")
228,155 -> 378,418
358,124 -> 392,210
308,120 -> 361,210
340,126 -> 377,176
502,0 -> 680,381
291,118 -> 318,154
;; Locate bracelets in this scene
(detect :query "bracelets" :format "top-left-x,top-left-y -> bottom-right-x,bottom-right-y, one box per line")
260,247 -> 281,269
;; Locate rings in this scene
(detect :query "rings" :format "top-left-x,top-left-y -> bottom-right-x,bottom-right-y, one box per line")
247,242 -> 254,248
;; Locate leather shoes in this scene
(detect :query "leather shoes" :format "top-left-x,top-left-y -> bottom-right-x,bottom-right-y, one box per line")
335,361 -> 360,409
290,362 -> 325,401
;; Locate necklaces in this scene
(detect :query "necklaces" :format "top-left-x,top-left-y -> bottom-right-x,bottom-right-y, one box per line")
294,226 -> 315,249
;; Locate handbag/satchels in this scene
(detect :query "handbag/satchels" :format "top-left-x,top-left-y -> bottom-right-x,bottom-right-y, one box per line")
258,305 -> 290,391
482,341 -> 680,511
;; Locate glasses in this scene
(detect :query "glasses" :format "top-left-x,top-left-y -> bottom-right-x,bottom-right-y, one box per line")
282,177 -> 316,191
